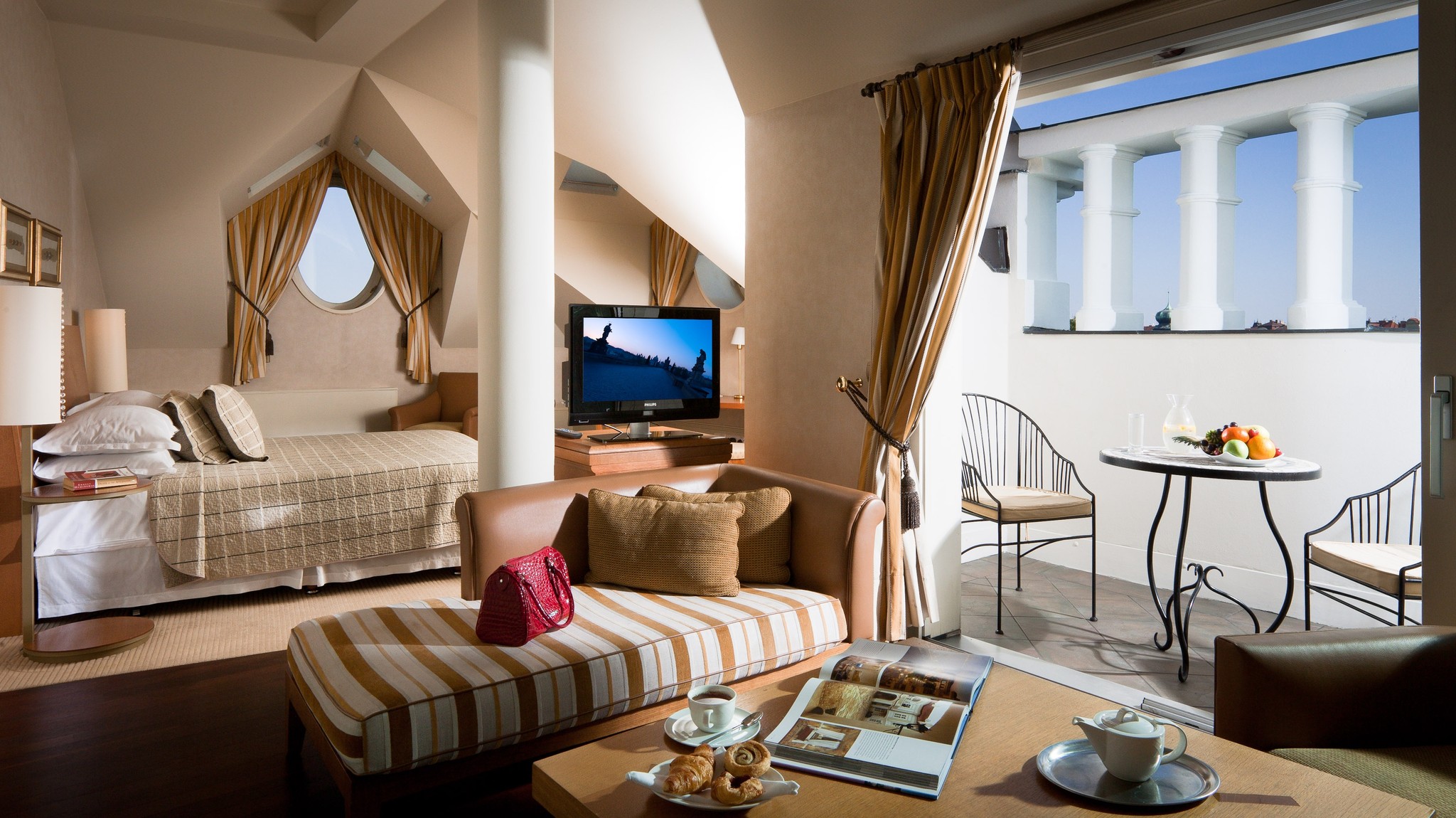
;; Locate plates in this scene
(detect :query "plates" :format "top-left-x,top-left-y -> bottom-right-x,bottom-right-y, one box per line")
1201,446 -> 1284,467
648,758 -> 785,810
664,707 -> 761,749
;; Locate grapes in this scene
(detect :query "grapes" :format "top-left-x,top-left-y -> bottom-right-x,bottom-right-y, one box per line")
1201,422 -> 1238,451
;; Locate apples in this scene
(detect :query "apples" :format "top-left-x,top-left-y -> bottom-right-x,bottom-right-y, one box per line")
1223,439 -> 1249,459
1247,425 -> 1269,437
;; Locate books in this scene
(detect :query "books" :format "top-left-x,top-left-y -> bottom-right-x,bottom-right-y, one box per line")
763,637 -> 995,801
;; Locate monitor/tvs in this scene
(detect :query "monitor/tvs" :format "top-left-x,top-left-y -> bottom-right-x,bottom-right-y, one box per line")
568,303 -> 721,442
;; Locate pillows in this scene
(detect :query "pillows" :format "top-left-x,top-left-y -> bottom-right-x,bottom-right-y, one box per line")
588,489 -> 740,596
32,383 -> 269,485
640,485 -> 792,586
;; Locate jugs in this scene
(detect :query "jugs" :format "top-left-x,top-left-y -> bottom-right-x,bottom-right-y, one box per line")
1071,707 -> 1188,782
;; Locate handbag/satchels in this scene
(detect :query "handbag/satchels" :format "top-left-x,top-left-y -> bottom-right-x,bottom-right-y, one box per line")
476,545 -> 575,647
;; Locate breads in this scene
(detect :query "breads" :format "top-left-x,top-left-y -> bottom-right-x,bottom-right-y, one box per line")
724,740 -> 771,778
711,771 -> 766,805
663,744 -> 715,795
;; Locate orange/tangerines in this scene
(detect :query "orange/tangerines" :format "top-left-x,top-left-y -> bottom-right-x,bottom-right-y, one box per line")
1222,427 -> 1249,443
1247,435 -> 1276,460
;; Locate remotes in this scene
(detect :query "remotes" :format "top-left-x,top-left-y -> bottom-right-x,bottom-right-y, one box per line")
555,428 -> 582,439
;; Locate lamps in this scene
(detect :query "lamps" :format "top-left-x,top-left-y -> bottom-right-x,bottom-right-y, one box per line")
731,327 -> 745,398
84,308 -> 128,394
0,284 -> 66,486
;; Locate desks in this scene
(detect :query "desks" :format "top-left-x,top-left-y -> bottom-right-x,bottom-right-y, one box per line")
1098,448 -> 1321,681
532,638 -> 1436,818
720,395 -> 744,409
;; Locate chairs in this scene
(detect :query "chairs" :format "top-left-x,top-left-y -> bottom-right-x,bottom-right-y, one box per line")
388,371 -> 478,439
1302,462 -> 1421,632
959,393 -> 1098,635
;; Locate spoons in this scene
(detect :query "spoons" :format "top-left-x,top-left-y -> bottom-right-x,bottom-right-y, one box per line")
701,711 -> 763,745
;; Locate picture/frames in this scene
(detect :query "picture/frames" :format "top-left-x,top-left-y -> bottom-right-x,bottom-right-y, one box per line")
0,198 -> 32,281
30,218 -> 63,286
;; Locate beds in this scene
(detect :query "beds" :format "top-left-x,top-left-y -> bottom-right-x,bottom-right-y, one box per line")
0,426 -> 479,639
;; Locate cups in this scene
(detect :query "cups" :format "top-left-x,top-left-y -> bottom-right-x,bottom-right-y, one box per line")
687,685 -> 737,733
1127,413 -> 1145,454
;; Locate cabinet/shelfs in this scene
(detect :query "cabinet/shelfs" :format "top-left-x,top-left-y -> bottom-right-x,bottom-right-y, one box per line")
554,428 -> 732,479
21,476 -> 155,657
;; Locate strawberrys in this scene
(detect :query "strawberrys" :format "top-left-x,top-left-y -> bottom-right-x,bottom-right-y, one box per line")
1214,446 -> 1222,455
1248,427 -> 1259,438
1274,448 -> 1282,458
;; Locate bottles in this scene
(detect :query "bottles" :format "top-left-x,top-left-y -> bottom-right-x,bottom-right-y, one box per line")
1163,393 -> 1197,455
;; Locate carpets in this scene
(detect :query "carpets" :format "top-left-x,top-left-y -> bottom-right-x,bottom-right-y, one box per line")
0,569 -> 462,692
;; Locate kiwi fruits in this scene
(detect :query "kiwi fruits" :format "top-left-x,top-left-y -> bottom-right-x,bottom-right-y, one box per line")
1206,430 -> 1221,443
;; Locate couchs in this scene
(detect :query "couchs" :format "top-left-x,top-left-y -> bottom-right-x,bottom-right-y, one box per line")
280,463 -> 885,818
1215,627 -> 1456,818
